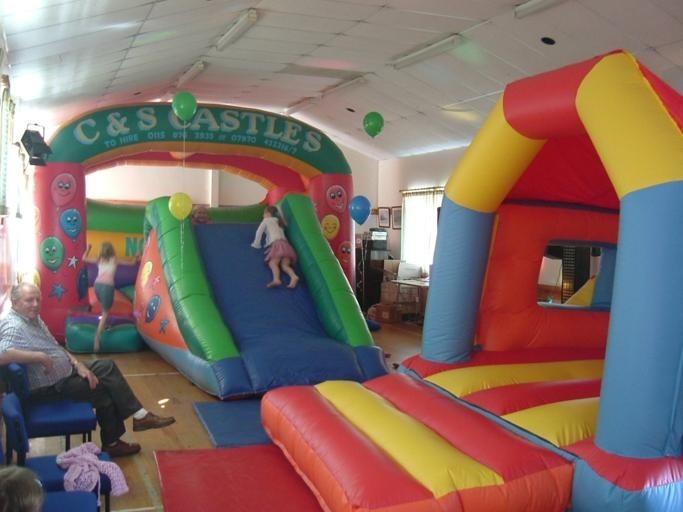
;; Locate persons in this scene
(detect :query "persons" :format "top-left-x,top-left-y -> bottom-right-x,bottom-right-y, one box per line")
0,465 -> 46,512
82,241 -> 139,353
251,206 -> 299,289
0,282 -> 176,458
191,205 -> 214,224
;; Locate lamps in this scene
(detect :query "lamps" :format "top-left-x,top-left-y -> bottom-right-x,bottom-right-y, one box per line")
176,59 -> 211,89
214,8 -> 258,51
514,1 -> 563,18
284,30 -> 470,115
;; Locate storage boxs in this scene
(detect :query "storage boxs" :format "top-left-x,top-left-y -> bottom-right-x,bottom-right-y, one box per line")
362,229 -> 430,324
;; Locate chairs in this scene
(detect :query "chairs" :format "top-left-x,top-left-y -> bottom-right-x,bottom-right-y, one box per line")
1,393 -> 113,511
5,361 -> 96,454
0,440 -> 97,511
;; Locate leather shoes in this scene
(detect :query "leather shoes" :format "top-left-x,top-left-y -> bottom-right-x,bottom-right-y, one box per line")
132,411 -> 177,432
101,439 -> 142,458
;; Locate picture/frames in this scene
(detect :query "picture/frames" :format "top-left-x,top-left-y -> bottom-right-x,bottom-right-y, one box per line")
392,205 -> 402,230
377,206 -> 390,228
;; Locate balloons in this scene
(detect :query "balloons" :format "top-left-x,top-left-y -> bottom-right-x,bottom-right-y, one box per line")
171,90 -> 197,121
349,195 -> 371,226
168,192 -> 193,221
362,111 -> 385,138
